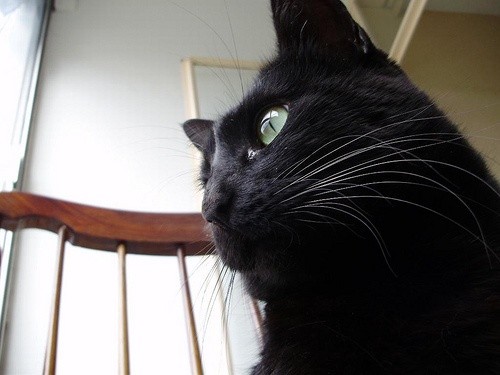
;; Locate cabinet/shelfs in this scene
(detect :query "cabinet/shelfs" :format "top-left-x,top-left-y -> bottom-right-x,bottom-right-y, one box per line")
341,0 -> 500,190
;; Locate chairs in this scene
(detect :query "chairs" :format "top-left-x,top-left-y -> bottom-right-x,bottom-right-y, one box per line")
1,187 -> 265,375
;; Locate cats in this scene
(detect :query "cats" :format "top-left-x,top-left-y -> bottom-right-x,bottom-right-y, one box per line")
182,0 -> 500,372
